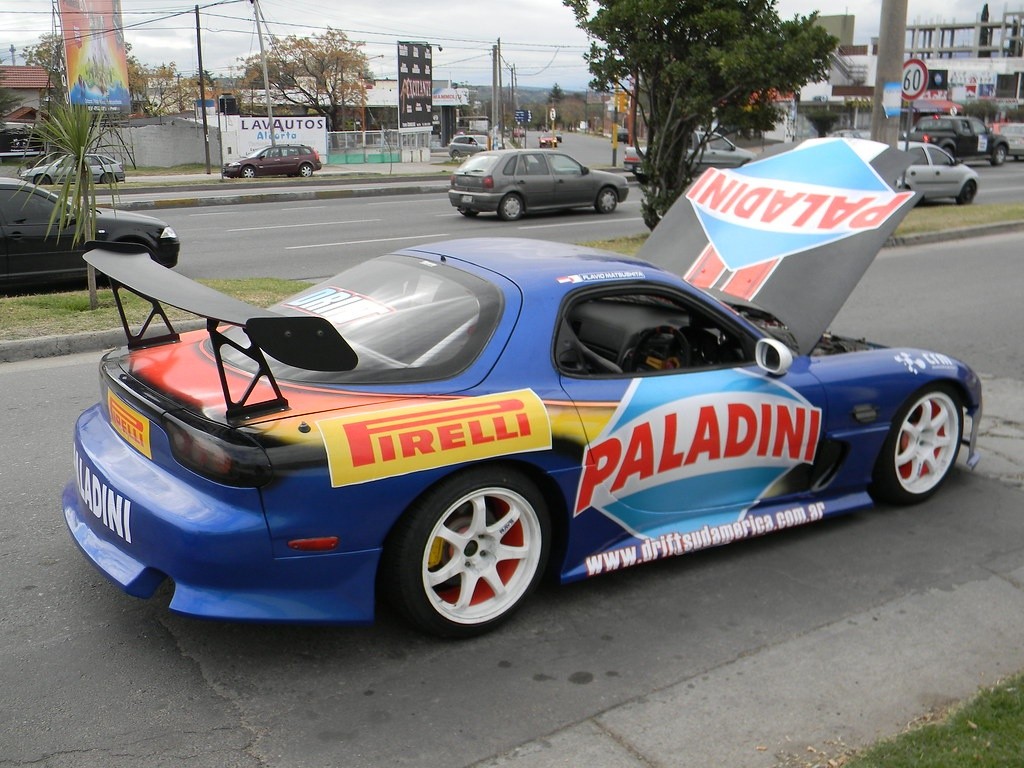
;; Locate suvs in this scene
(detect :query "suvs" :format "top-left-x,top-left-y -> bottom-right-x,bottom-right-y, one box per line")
222,143 -> 322,178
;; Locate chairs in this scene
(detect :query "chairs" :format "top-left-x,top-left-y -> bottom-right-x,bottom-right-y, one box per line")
273,151 -> 279,157
432,283 -> 466,304
530,162 -> 540,175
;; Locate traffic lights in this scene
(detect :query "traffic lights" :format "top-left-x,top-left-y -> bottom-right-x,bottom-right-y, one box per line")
620,92 -> 627,113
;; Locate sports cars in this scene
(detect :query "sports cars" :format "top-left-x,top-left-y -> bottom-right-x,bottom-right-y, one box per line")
62,138 -> 984,643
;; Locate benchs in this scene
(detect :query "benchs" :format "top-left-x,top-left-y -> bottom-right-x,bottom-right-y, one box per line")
287,151 -> 296,156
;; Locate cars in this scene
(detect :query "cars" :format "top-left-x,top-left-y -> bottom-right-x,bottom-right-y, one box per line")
827,130 -> 871,139
548,130 -> 562,143
1002,123 -> 1024,160
448,135 -> 507,158
19,153 -> 125,185
0,177 -> 180,289
448,148 -> 629,222
896,140 -> 981,206
538,133 -> 558,148
611,128 -> 629,144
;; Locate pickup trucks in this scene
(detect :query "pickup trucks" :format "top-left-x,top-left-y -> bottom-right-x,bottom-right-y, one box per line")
899,114 -> 1010,167
623,131 -> 756,185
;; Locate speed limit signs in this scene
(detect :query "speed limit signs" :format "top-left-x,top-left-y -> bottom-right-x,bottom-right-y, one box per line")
901,59 -> 929,102
